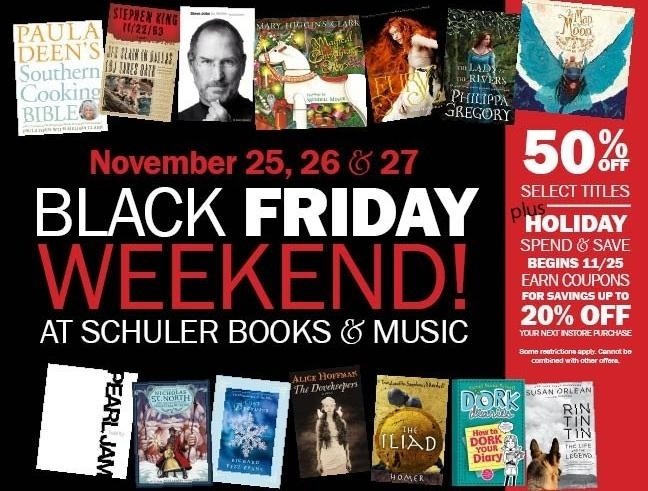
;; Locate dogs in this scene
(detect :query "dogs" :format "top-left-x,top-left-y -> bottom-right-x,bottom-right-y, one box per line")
527,438 -> 561,491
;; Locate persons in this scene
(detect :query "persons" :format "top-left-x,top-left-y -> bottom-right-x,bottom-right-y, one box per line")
315,397 -> 352,477
175,18 -> 252,121
163,442 -> 181,478
368,18 -> 438,123
156,426 -> 193,479
465,30 -> 508,108
